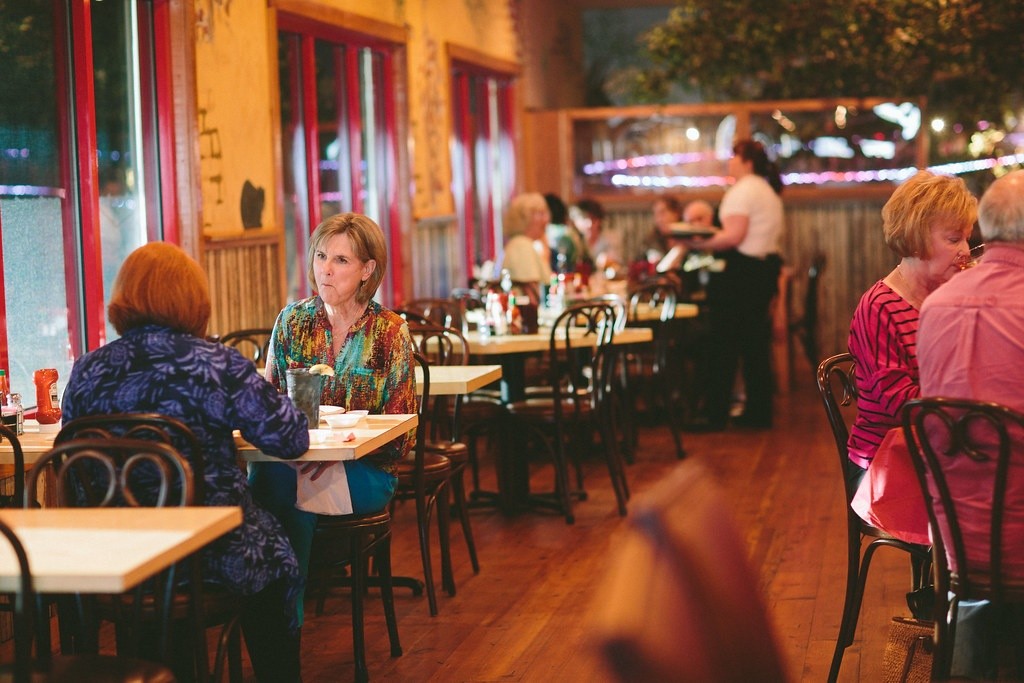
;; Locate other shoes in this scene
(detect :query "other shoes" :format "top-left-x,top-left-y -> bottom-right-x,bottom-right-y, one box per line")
684,419 -> 726,432
730,414 -> 770,429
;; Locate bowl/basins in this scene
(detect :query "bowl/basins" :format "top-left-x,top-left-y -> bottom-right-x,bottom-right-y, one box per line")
318,405 -> 369,429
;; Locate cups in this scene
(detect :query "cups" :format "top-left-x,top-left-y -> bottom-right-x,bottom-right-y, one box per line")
284,367 -> 322,430
956,243 -> 984,270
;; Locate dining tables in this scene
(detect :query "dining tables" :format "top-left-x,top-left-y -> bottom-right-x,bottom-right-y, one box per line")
0,409 -> 421,466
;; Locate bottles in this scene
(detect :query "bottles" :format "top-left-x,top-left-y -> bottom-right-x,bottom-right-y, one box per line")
0,370 -> 9,406
33,368 -> 62,433
484,271 -> 578,337
6,393 -> 23,435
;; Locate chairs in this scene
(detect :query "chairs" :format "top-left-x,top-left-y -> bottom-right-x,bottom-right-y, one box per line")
814,350 -> 1024,683
0,274 -> 688,683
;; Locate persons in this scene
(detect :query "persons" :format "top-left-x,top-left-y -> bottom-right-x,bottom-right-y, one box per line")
503,190 -> 549,305
849,166 -> 976,470
543,192 -> 729,303
63,240 -> 310,683
247,210 -> 417,629
681,136 -> 787,432
916,169 -> 1024,584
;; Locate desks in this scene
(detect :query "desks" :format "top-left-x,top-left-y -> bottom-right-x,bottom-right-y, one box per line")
1,504 -> 245,650
535,304 -> 701,459
256,364 -> 503,400
411,326 -> 653,511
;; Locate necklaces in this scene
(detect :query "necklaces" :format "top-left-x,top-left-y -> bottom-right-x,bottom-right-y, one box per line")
897,265 -> 922,305
333,331 -> 348,340
